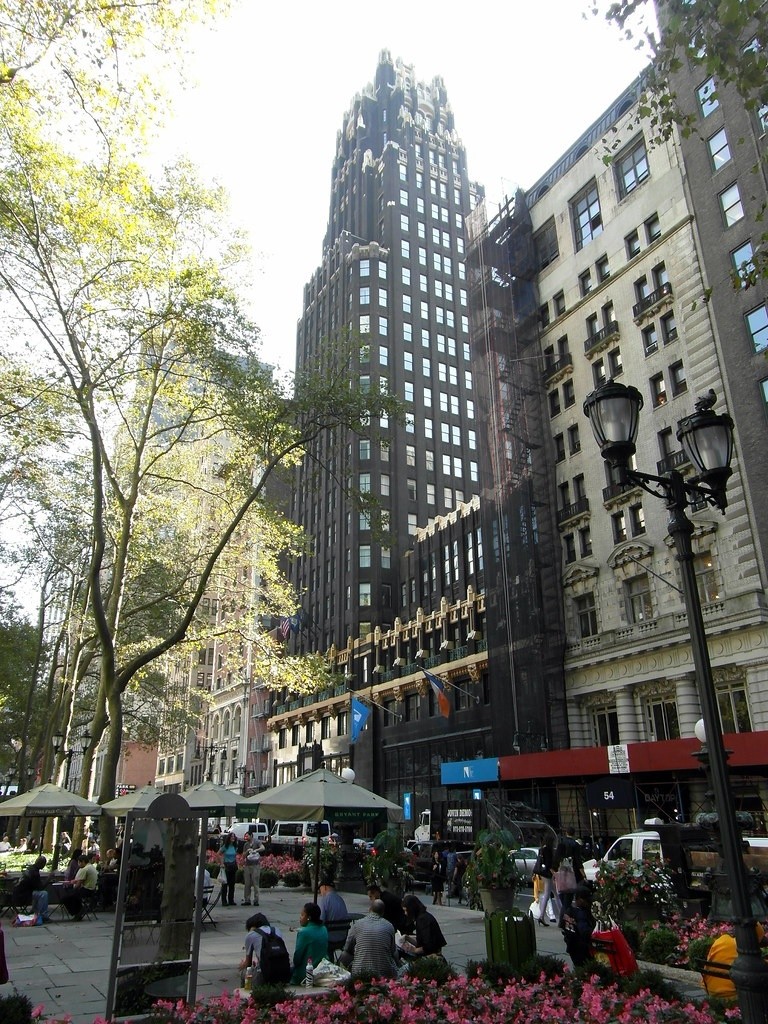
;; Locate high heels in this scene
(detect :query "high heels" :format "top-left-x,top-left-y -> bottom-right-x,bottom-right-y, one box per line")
538,918 -> 550,927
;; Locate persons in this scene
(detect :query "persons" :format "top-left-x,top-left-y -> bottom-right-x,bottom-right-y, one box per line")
365,885 -> 417,935
701,921 -> 765,1002
15,856 -> 52,923
532,826 -> 624,928
316,878 -> 351,921
290,903 -> 329,984
235,912 -> 291,990
342,899 -> 399,980
0,835 -> 28,855
60,819 -> 266,909
312,821 -> 480,910
396,894 -> 446,968
62,855 -> 98,922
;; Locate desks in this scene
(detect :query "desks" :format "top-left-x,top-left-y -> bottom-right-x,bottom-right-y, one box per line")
48,881 -> 74,920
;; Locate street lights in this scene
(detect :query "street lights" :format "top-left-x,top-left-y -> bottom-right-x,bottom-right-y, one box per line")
195,738 -> 228,783
25,762 -> 34,791
582,377 -> 768,1024
51,728 -> 93,871
233,763 -> 255,823
690,717 -> 768,923
334,767 -> 365,892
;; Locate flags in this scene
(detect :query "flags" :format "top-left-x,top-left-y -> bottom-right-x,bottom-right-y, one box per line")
281,602 -> 305,643
422,668 -> 450,719
351,695 -> 371,744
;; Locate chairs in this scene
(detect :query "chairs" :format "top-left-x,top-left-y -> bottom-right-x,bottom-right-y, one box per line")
199,883 -> 227,931
324,917 -> 355,963
0,863 -> 104,922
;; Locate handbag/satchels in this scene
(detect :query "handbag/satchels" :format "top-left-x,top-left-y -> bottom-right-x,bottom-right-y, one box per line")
217,868 -> 228,884
551,858 -> 578,896
301,958 -> 352,988
590,915 -> 640,979
532,848 -> 546,877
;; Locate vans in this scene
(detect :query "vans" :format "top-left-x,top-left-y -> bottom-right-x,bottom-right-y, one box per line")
266,820 -> 331,846
222,822 -> 269,844
601,818 -> 768,902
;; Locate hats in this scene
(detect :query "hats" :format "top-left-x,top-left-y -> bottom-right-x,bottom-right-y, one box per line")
318,878 -> 335,889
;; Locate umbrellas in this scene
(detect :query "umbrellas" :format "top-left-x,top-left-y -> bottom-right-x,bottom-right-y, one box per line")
104,769 -> 248,820
236,767 -> 405,903
0,783 -> 102,818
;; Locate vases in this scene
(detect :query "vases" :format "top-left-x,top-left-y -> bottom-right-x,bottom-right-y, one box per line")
623,904 -> 660,922
308,868 -> 328,893
479,889 -> 515,915
379,876 -> 406,897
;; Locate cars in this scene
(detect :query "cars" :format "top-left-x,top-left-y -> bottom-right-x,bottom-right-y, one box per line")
508,848 -> 600,885
349,838 -> 416,879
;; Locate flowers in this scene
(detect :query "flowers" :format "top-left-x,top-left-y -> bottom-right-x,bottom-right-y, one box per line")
589,854 -> 688,919
464,828 -> 526,898
302,835 -> 338,885
363,830 -> 415,887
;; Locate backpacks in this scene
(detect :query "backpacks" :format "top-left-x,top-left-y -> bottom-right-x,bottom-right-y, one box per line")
252,927 -> 291,985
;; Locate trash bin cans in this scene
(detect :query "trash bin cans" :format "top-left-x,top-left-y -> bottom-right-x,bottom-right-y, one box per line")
484,906 -> 536,966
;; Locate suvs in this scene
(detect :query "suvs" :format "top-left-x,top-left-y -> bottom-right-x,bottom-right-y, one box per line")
411,841 -> 475,885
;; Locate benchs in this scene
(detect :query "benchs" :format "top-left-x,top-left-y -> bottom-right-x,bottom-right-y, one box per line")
234,981 -> 333,999
560,928 -> 622,984
693,956 -> 731,995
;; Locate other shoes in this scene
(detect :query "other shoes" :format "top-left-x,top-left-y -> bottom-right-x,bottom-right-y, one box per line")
228,902 -> 237,906
241,902 -> 251,906
254,902 -> 259,906
222,903 -> 230,906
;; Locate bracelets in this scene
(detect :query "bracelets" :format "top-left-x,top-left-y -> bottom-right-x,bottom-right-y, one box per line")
583,875 -> 587,878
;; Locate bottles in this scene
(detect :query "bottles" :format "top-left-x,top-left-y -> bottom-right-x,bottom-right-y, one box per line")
306,958 -> 313,989
245,967 -> 252,989
